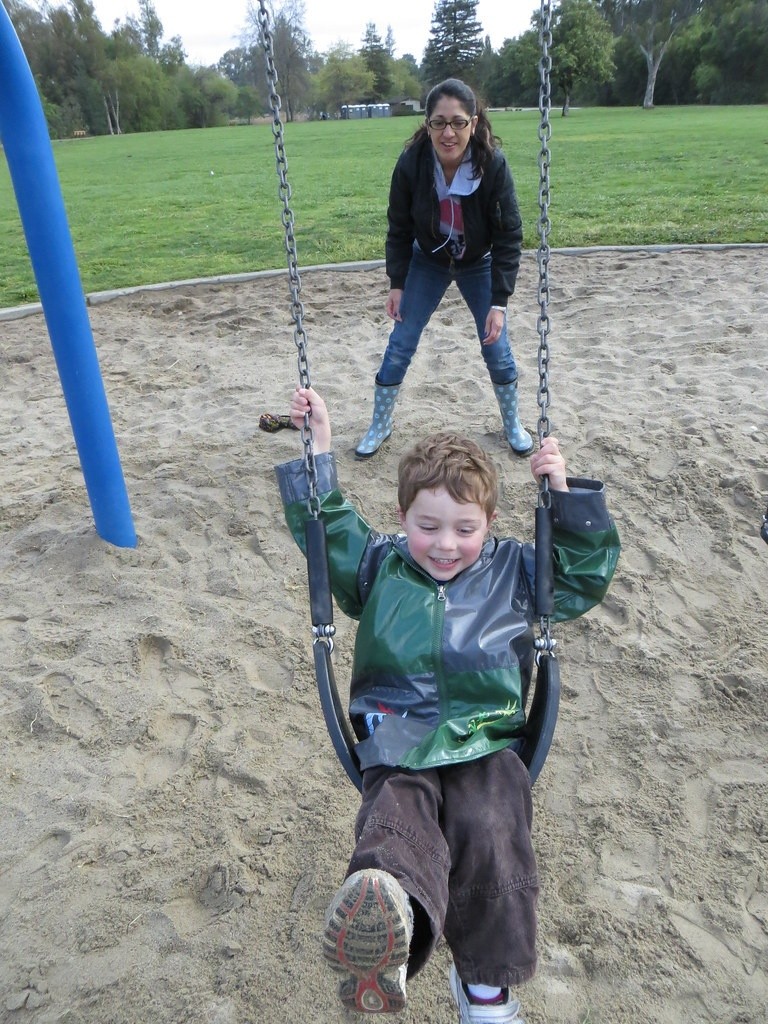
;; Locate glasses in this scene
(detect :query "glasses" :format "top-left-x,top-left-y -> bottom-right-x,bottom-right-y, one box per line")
426,115 -> 473,129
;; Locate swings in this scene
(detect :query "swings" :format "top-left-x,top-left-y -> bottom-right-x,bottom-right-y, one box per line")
248,0 -> 566,798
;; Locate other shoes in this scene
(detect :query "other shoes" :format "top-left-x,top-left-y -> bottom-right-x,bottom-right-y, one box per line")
321,869 -> 412,1012
449,962 -> 519,1024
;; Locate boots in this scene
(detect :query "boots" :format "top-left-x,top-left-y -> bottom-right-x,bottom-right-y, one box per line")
491,379 -> 535,458
355,373 -> 402,457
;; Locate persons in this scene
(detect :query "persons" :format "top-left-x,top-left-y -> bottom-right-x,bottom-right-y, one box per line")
355,78 -> 535,456
287,382 -> 618,1024
